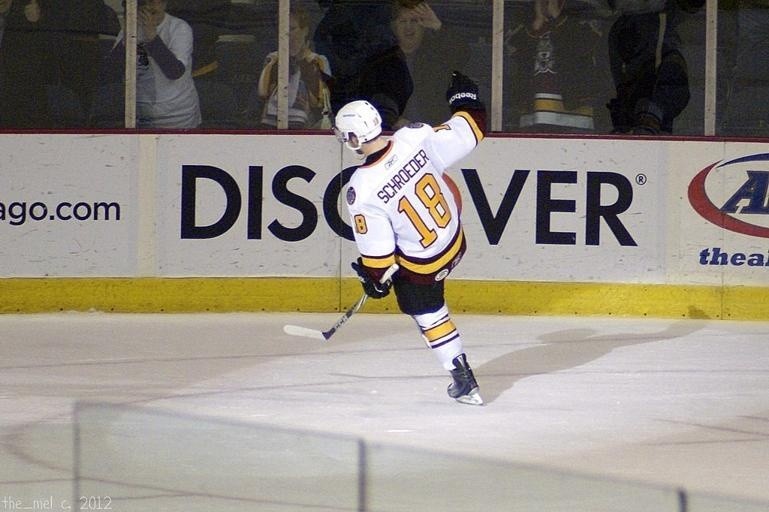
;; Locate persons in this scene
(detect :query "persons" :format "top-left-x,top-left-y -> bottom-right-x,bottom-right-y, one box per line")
334,69 -> 486,399
110,0 -> 201,129
367,0 -> 474,130
257,9 -> 331,130
505,0 -> 595,132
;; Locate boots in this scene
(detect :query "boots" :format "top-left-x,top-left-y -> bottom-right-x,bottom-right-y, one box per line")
447,354 -> 478,399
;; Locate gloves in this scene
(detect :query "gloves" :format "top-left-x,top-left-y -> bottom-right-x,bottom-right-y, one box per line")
446,72 -> 485,110
351,257 -> 389,298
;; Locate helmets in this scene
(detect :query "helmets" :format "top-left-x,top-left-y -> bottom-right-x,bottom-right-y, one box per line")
335,101 -> 382,143
